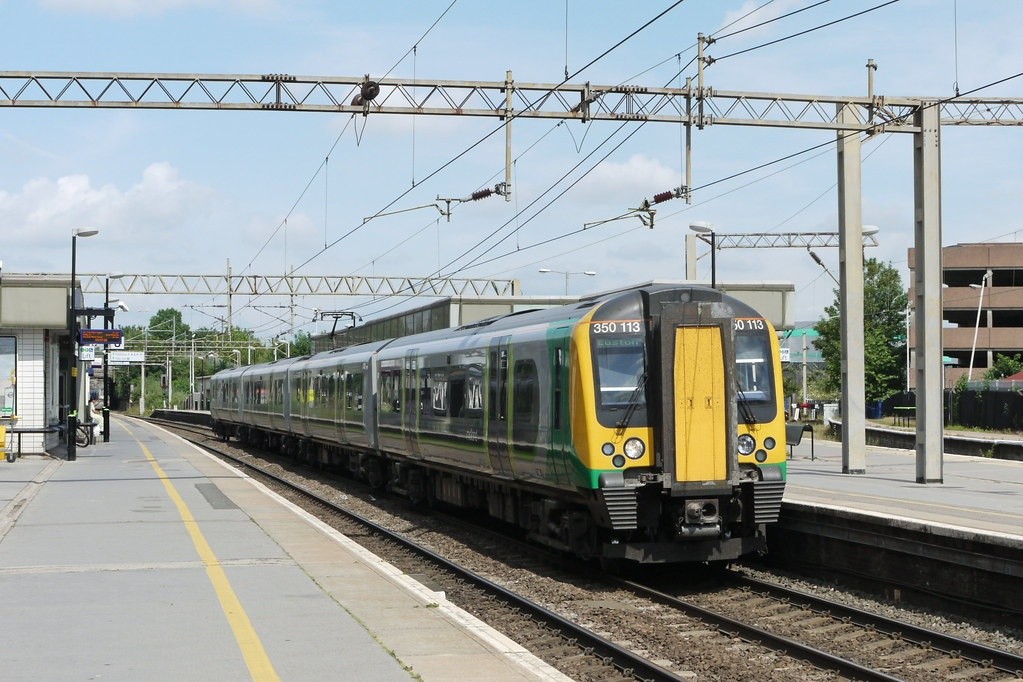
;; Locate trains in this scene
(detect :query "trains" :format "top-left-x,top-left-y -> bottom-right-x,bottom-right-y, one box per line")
210,284 -> 787,563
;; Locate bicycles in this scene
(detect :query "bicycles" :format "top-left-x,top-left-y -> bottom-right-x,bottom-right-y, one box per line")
61,419 -> 89,448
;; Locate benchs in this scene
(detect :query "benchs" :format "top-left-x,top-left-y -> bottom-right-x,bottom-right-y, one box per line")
5,425 -> 67,458
79,422 -> 98,445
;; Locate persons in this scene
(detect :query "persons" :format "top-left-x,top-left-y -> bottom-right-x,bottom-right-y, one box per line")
88,395 -> 104,435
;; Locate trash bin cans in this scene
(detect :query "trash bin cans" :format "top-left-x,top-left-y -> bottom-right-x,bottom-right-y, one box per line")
865,398 -> 884,419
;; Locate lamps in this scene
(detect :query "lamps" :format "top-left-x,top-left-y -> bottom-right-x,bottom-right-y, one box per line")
111,302 -> 129,312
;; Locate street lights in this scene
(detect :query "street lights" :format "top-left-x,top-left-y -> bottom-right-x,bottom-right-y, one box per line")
103,271 -> 125,442
249,346 -> 255,364
198,357 -> 204,410
232,350 -> 241,367
138,309 -> 148,339
279,340 -> 290,357
539,269 -> 597,297
192,333 -> 196,410
68,227 -> 99,461
690,223 -> 714,291
